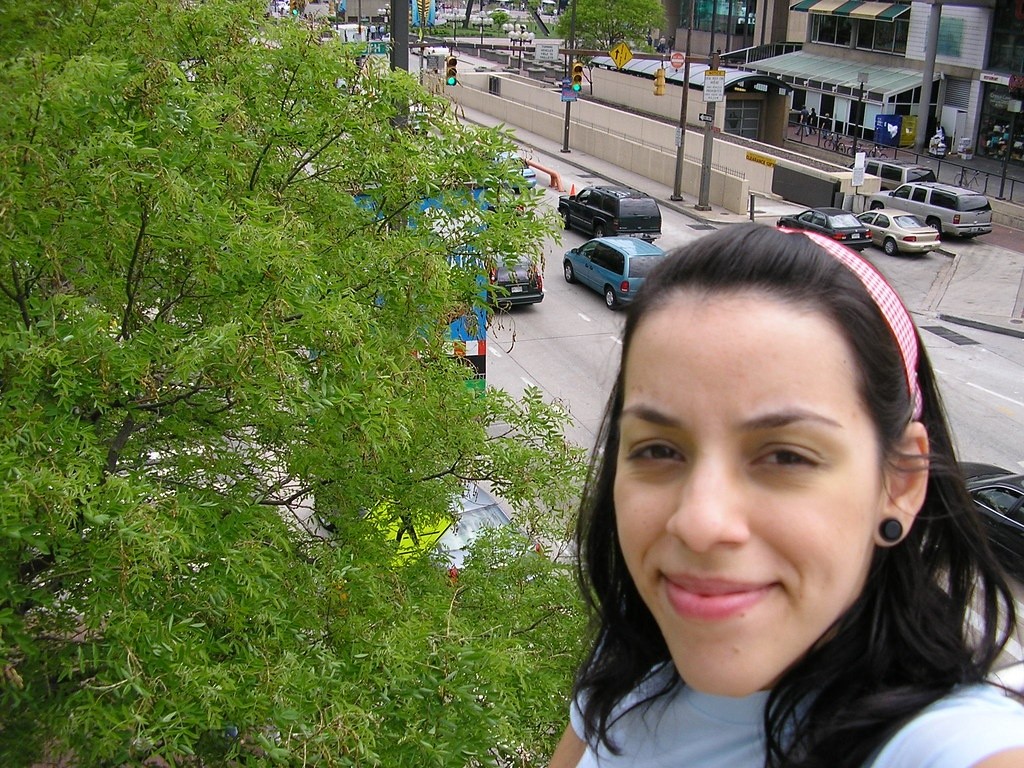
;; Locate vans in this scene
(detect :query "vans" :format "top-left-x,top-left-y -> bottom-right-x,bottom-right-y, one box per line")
843,157 -> 937,190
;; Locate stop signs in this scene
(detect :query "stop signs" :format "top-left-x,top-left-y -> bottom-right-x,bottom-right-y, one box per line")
671,53 -> 685,68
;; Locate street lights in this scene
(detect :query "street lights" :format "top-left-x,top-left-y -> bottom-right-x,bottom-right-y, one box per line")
851,72 -> 869,156
995,99 -> 1022,200
508,25 -> 535,74
444,12 -> 466,50
471,11 -> 494,45
377,4 -> 391,34
502,20 -> 527,57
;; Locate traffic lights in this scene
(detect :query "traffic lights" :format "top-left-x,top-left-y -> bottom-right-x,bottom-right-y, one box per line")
572,63 -> 583,93
444,55 -> 458,86
289,0 -> 298,15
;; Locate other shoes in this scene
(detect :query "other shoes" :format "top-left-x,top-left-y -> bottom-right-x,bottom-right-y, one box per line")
794,132 -> 799,135
806,135 -> 808,137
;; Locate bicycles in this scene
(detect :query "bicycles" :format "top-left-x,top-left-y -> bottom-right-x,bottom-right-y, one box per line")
953,168 -> 982,192
822,132 -> 889,159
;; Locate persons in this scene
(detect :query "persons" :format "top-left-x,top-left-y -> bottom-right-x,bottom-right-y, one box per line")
809,107 -> 817,135
795,105 -> 809,136
822,113 -> 831,139
548,224 -> 1024,768
668,35 -> 674,54
658,35 -> 665,46
717,47 -> 721,55
931,125 -> 945,143
645,31 -> 652,46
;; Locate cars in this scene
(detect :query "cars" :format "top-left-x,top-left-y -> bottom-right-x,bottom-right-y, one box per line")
487,190 -> 524,219
491,152 -> 538,193
562,235 -> 672,311
855,208 -> 942,256
485,252 -> 544,308
775,206 -> 874,252
952,461 -> 1024,584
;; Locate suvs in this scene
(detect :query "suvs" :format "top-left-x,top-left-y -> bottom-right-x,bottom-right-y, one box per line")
557,185 -> 662,244
868,182 -> 993,241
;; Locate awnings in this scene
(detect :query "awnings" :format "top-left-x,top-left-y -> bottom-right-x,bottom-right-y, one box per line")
789,0 -> 911,23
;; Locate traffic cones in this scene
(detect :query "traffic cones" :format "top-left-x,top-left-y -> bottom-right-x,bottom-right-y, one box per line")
570,183 -> 576,195
